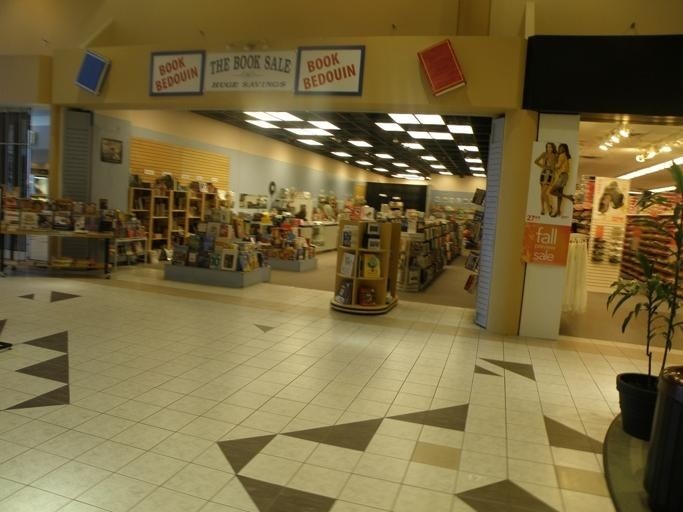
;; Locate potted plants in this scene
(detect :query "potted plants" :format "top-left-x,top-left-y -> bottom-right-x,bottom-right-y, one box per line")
628,158 -> 683,511
603,255 -> 661,442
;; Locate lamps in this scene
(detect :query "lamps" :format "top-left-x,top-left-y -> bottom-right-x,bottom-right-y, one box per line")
597,127 -> 630,152
635,136 -> 683,163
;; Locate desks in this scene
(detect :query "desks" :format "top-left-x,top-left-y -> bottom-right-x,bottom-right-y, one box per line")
113,238 -> 147,266
0,222 -> 112,282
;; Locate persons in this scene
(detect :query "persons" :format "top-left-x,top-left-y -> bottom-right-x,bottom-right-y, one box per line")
549,144 -> 574,217
534,143 -> 558,215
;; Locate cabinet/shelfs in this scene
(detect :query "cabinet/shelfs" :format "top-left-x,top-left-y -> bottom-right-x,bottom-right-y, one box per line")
332,219 -> 398,314
130,188 -> 231,261
572,178 -> 631,296
622,213 -> 682,298
397,219 -> 459,292
